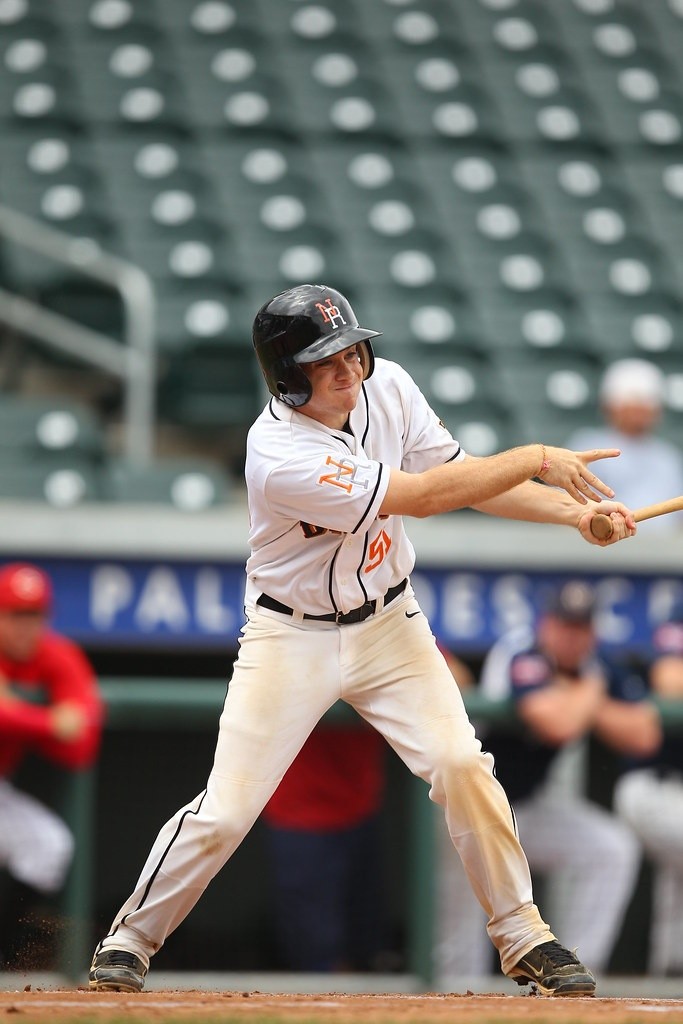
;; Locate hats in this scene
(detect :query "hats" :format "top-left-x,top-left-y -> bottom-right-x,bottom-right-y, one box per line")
0,565 -> 53,614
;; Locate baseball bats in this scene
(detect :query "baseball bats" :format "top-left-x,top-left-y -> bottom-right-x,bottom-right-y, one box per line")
589,494 -> 683,542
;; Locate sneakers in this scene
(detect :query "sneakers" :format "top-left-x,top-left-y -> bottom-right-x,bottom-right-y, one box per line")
506,941 -> 595,998
88,950 -> 147,992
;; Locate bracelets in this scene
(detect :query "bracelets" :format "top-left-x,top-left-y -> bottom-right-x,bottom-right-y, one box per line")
537,443 -> 550,475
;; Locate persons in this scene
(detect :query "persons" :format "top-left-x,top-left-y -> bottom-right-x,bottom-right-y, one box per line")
87,282 -> 639,996
263,645 -> 473,968
481,600 -> 659,973
0,562 -> 105,894
614,625 -> 683,974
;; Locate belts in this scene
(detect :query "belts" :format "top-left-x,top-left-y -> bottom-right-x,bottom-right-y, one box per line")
259,579 -> 409,624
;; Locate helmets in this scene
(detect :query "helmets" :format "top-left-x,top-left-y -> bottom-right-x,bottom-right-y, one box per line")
252,283 -> 377,409
